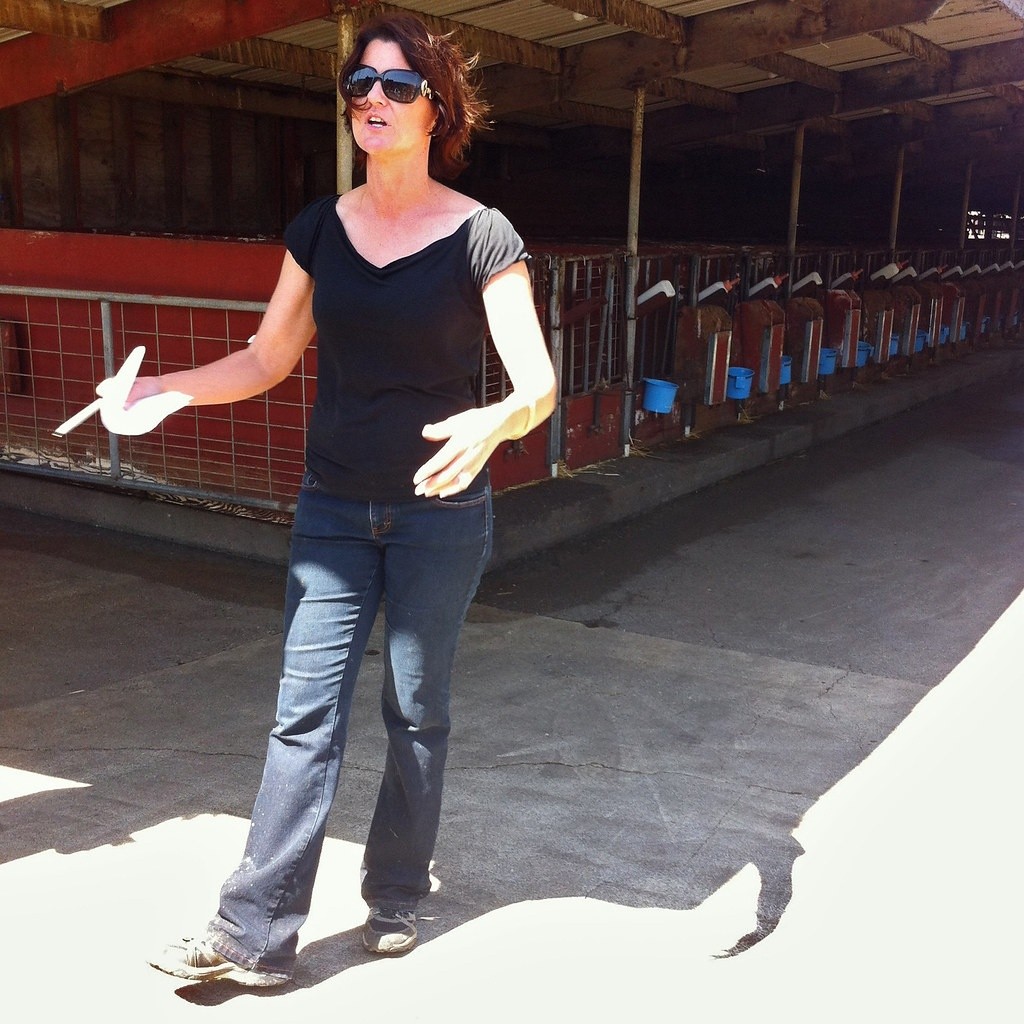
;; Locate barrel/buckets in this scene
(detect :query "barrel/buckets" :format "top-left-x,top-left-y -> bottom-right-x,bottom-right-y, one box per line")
780,356 -> 792,384
726,367 -> 755,399
940,327 -> 948,344
889,338 -> 898,356
961,321 -> 967,340
818,348 -> 837,375
642,378 -> 679,414
856,341 -> 872,368
915,329 -> 927,352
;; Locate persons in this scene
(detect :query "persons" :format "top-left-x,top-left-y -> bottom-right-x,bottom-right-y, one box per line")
97,13 -> 556,987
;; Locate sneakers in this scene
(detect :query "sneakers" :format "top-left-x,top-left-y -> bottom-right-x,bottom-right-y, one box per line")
146,935 -> 289,987
362,907 -> 418,953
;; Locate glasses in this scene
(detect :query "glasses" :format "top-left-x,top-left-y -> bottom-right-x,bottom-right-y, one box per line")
342,63 -> 448,107
